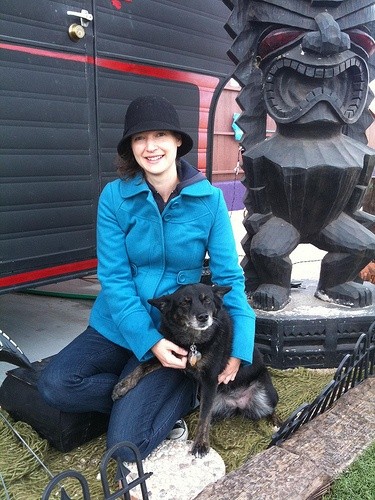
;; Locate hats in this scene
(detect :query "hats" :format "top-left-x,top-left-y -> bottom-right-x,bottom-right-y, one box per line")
117,95 -> 193,156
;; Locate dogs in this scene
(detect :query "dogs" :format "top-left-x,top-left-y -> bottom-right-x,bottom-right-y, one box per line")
112,283 -> 284,457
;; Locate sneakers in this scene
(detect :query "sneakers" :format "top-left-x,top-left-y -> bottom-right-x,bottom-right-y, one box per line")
166,417 -> 189,442
